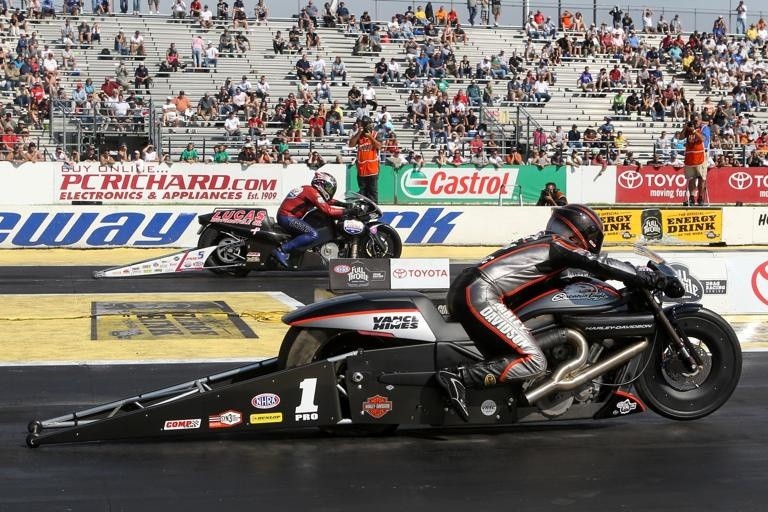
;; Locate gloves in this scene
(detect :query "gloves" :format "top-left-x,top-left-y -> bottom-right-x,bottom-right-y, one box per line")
342,206 -> 360,217
336,199 -> 354,209
636,270 -> 667,292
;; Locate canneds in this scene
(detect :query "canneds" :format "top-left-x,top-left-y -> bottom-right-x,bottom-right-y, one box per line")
641,209 -> 662,241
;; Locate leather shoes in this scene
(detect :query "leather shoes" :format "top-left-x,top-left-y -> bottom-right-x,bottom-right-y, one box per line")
271,247 -> 289,269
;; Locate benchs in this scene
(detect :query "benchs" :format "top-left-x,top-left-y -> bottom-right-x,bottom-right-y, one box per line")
0,6 -> 768,173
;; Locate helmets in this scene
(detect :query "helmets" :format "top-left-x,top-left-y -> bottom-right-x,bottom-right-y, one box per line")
546,203 -> 605,255
311,170 -> 338,202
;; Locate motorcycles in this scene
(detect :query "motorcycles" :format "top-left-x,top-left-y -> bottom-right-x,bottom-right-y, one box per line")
273,237 -> 744,421
195,190 -> 402,280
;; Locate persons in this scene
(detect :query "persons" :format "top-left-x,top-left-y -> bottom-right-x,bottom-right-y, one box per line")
678,113 -> 711,205
348,115 -> 382,205
0,0 -> 768,173
439,205 -> 686,422
536,181 -> 568,206
270,171 -> 362,270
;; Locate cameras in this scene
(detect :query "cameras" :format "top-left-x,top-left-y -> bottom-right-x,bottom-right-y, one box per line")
687,121 -> 695,127
542,189 -> 554,197
361,120 -> 369,137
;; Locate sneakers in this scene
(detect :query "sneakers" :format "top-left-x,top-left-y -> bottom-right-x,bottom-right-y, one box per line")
434,364 -> 470,424
